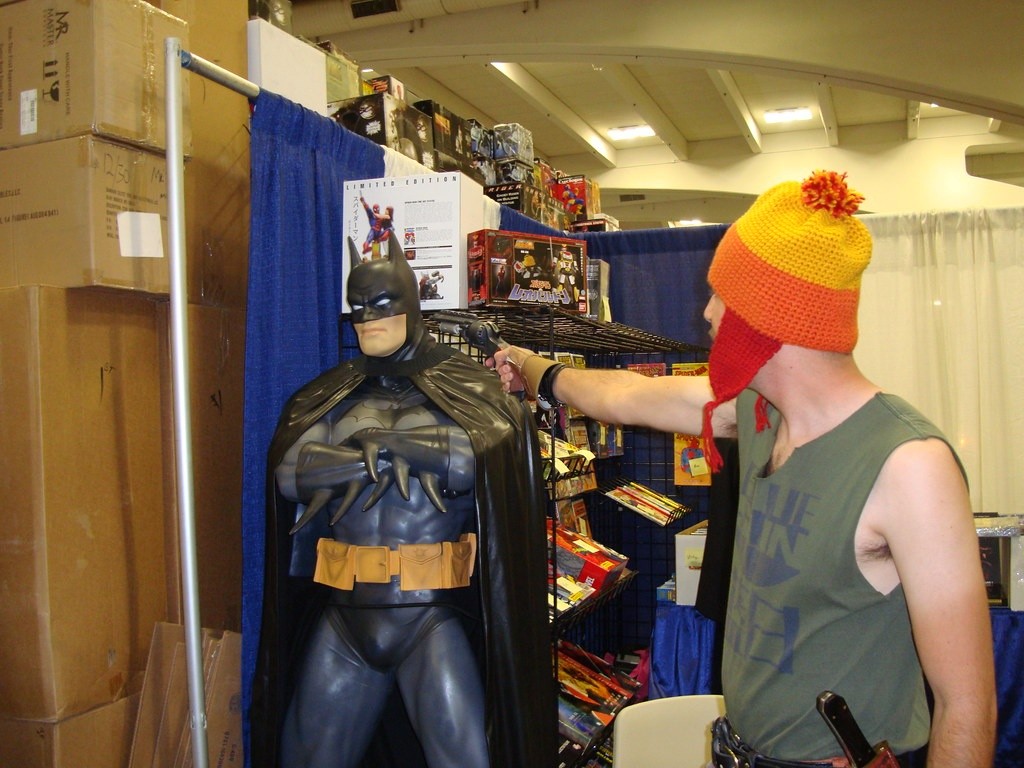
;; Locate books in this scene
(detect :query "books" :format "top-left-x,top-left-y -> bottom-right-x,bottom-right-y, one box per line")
539,350 -> 708,768
974,512 -> 1024,613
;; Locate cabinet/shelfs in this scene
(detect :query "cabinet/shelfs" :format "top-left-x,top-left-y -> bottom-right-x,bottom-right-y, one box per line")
338,299 -> 710,768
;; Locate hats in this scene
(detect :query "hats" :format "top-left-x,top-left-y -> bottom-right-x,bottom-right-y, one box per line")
699,168 -> 873,475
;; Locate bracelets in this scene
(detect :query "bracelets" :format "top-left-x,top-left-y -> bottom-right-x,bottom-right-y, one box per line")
537,363 -> 565,409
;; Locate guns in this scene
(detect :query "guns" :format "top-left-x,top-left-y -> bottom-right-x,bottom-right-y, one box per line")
421,306 -> 527,404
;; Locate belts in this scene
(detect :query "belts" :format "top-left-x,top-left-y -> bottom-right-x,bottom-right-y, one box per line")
711,713 -> 930,768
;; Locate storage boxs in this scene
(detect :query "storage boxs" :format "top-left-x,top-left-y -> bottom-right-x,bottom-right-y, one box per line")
247,15 -> 650,768
1,0 -> 244,767
978,535 -> 1011,607
657,518 -> 710,609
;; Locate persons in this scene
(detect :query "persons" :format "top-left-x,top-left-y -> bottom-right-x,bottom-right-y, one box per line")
485,173 -> 999,767
254,229 -> 545,768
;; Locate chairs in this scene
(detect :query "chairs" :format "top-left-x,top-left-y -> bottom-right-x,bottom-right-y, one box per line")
612,694 -> 728,768
